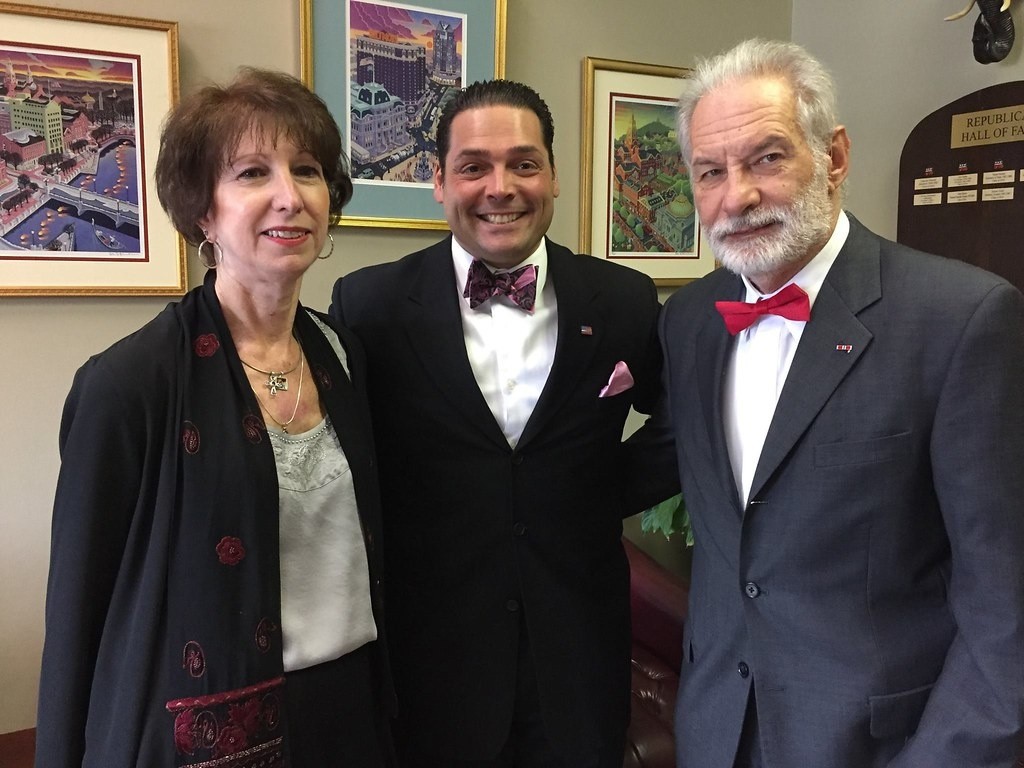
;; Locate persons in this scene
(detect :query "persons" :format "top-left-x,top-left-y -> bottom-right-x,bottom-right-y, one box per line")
33,71 -> 399,768
622,39 -> 1024,768
328,78 -> 683,768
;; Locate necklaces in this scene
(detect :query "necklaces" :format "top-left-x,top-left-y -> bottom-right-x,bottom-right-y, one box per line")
246,328 -> 304,434
240,326 -> 301,396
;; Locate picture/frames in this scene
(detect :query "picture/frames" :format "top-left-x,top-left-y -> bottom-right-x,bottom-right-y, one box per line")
299,0 -> 508,231
0,2 -> 189,298
579,55 -> 720,287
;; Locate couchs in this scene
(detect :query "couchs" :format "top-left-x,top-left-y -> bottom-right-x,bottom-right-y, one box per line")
0,536 -> 690,768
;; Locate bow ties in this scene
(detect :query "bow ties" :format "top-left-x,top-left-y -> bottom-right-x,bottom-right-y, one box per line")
462,261 -> 540,315
714,282 -> 812,338
